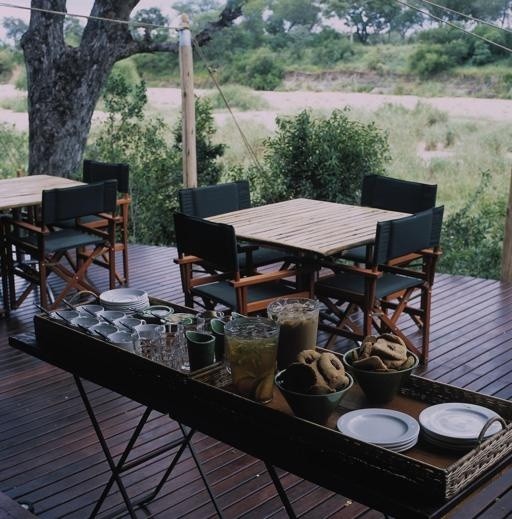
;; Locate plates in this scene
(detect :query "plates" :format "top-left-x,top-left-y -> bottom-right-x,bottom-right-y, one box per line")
99,288 -> 151,313
335,408 -> 419,452
418,403 -> 503,450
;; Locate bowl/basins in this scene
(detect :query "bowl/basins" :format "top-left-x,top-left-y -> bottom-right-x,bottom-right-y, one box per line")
272,365 -> 353,427
347,344 -> 415,400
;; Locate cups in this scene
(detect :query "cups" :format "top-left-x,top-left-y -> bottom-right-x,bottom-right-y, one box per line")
48,306 -> 245,369
268,296 -> 325,383
224,315 -> 281,406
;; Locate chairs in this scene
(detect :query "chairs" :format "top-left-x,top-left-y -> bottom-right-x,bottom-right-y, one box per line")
319,170 -> 440,329
57,158 -> 129,290
177,180 -> 293,285
317,206 -> 449,363
1,179 -> 118,314
170,209 -> 319,320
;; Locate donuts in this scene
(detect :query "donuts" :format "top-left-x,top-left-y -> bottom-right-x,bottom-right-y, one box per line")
283,349 -> 349,394
351,333 -> 415,372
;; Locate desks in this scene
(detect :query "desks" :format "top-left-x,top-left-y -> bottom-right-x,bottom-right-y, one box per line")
7,291 -> 512,519
2,174 -> 88,302
203,195 -> 415,297
